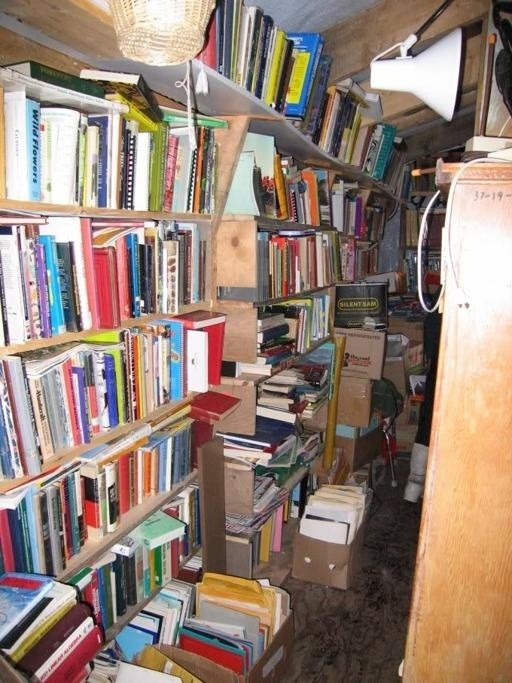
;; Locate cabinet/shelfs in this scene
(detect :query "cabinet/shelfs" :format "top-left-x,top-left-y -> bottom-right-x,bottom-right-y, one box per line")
401,155 -> 512,683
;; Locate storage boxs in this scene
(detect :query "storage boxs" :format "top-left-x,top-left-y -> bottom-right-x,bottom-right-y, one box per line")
330,282 -> 391,473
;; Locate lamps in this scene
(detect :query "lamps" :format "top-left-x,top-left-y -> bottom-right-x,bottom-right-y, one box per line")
365,0 -> 468,127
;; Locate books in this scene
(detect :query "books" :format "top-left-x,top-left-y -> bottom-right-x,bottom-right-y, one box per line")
2,211 -> 207,347
404,156 -> 441,294
219,133 -> 387,579
195,3 -> 408,197
3,60 -> 231,213
2,310 -> 292,681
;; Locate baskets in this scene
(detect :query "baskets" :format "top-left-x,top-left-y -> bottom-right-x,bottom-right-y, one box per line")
107,1 -> 216,67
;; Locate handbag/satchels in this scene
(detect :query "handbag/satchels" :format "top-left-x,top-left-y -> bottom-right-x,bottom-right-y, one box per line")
371,378 -> 404,459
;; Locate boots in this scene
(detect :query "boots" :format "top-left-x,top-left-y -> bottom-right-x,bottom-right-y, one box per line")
403,442 -> 429,502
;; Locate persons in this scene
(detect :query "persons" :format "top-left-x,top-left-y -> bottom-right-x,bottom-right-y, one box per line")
403,281 -> 443,504
